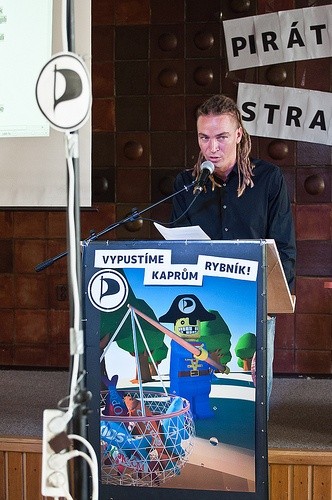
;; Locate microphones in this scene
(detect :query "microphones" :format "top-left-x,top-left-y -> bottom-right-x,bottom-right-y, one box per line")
195,160 -> 215,195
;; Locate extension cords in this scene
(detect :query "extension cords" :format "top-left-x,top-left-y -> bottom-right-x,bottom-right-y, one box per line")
42,409 -> 69,497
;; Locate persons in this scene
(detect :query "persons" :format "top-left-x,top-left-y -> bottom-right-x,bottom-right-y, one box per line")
170,92 -> 300,429
